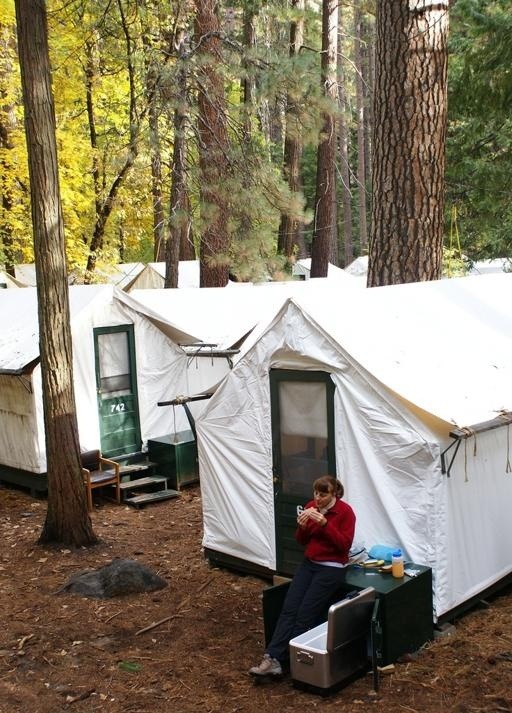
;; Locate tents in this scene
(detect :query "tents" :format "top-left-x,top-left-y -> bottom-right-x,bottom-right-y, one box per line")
2,249 -> 511,629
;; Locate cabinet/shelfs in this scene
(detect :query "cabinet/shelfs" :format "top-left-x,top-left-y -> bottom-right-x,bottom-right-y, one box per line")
263,557 -> 435,692
147,429 -> 199,491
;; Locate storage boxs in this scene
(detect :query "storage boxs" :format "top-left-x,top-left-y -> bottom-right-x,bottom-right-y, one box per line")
288,585 -> 375,699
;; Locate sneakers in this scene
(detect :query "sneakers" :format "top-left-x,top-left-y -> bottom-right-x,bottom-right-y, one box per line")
248,651 -> 284,685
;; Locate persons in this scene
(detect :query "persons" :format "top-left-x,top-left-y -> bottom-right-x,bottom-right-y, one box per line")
247,474 -> 357,686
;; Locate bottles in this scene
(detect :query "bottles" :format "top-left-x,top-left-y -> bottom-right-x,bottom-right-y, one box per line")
391,549 -> 404,578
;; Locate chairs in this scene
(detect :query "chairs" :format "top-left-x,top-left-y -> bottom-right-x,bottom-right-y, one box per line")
80,450 -> 119,512
